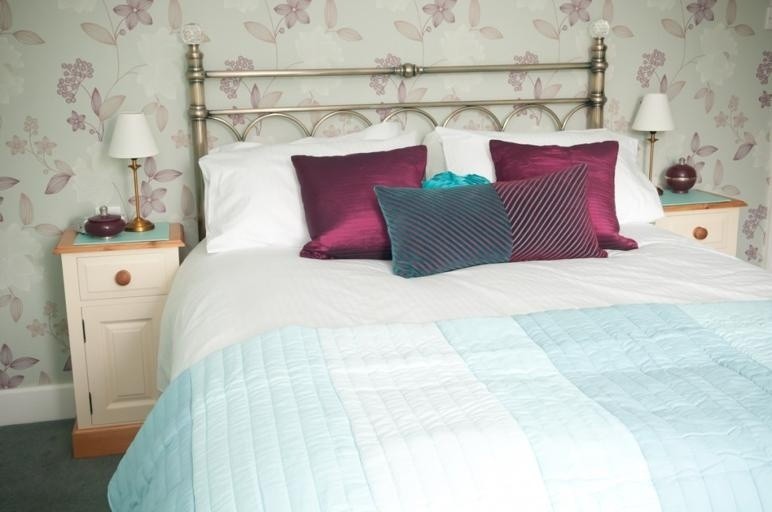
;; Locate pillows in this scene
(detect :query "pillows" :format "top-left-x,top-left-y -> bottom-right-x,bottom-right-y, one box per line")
491,163 -> 607,261
375,182 -> 511,279
197,129 -> 422,256
291,144 -> 428,261
423,129 -> 664,227
489,140 -> 639,250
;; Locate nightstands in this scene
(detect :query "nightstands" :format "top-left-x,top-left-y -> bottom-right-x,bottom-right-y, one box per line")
54,223 -> 186,460
658,189 -> 748,259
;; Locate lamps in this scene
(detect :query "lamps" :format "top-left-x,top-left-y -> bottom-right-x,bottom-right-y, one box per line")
632,93 -> 677,196
110,112 -> 160,233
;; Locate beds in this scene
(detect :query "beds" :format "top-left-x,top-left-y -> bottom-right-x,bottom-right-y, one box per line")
181,19 -> 772,512
